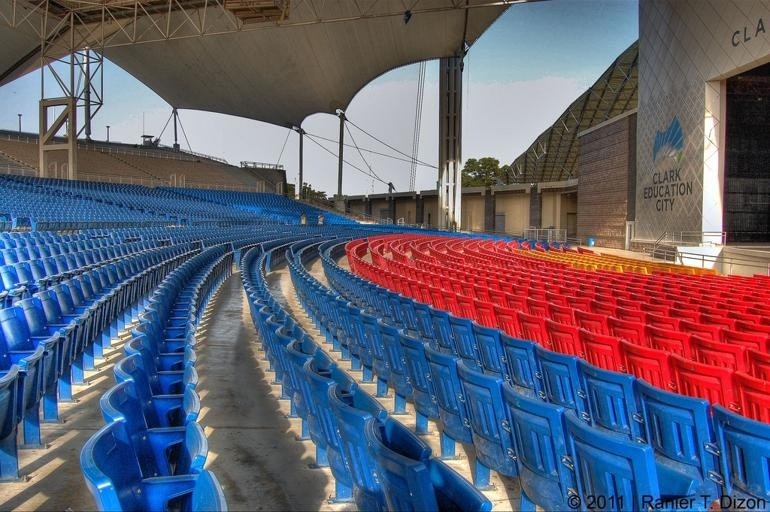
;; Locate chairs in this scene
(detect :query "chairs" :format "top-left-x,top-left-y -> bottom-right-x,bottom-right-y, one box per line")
1,176 -> 770,512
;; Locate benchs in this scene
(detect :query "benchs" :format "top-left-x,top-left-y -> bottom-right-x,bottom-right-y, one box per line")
0,136 -> 280,193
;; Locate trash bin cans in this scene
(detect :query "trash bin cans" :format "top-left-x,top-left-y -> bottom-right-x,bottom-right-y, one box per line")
586,238 -> 593,247
